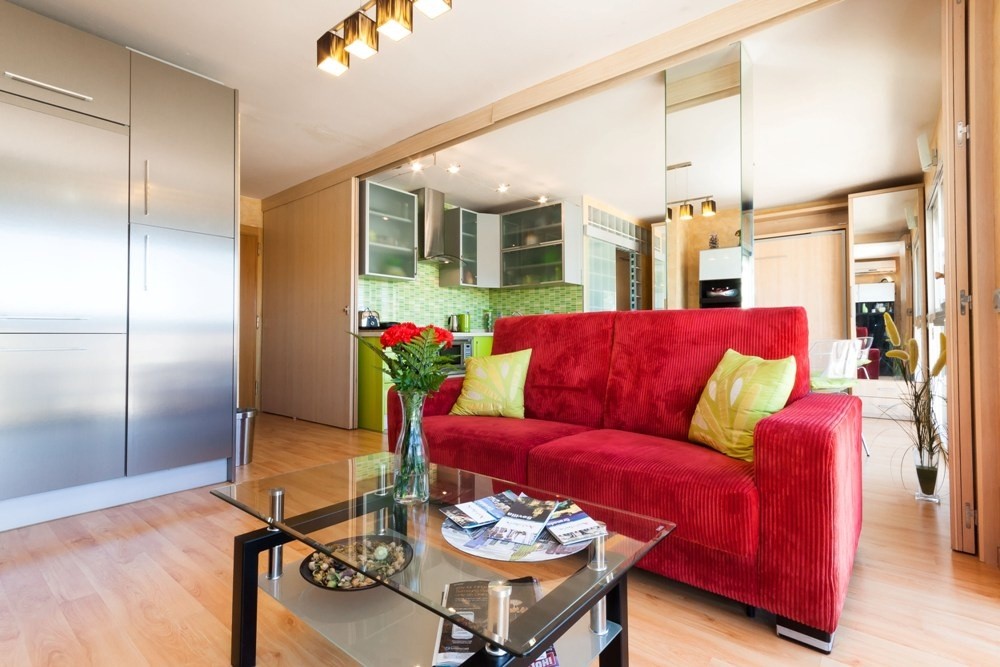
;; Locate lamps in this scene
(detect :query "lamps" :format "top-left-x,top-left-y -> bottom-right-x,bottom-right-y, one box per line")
905,206 -> 918,229
665,195 -> 716,223
316,0 -> 452,76
917,133 -> 937,173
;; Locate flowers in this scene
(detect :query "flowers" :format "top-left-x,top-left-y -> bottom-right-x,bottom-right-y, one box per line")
344,323 -> 454,497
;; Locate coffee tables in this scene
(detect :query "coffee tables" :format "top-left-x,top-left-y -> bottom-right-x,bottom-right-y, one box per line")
209,451 -> 676,667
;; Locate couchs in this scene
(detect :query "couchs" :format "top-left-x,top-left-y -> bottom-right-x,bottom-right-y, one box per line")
856,327 -> 880,379
386,307 -> 862,655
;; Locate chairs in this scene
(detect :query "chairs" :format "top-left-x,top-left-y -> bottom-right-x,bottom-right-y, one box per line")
857,336 -> 873,379
807,339 -> 870,458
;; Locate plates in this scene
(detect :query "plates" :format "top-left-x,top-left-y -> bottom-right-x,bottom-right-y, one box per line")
300,535 -> 413,591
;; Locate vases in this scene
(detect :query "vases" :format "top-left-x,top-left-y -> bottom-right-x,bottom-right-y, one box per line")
913,448 -> 941,504
393,391 -> 430,505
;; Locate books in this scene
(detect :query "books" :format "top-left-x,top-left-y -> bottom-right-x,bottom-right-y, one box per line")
438,490 -> 520,528
546,499 -> 609,545
487,491 -> 559,546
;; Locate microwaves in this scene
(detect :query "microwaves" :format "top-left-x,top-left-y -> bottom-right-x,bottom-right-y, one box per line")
424,340 -> 472,370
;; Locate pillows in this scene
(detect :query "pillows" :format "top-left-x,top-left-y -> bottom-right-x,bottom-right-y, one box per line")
447,349 -> 532,419
687,348 -> 796,463
859,338 -> 867,349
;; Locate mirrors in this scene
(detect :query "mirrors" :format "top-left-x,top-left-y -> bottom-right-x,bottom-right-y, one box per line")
665,41 -> 756,310
848,184 -> 928,383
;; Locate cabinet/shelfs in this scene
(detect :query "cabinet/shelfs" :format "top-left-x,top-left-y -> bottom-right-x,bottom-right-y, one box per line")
630,253 -> 642,311
358,180 -> 419,282
477,213 -> 500,288
471,337 -> 493,357
358,337 -> 412,433
439,207 -> 478,289
500,201 -> 583,289
